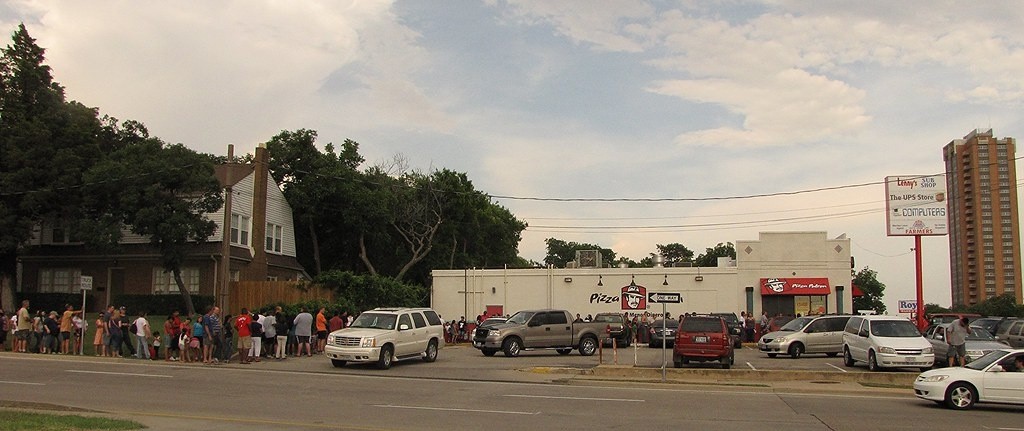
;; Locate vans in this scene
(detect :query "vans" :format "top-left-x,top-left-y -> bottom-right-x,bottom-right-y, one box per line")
928,313 -> 982,328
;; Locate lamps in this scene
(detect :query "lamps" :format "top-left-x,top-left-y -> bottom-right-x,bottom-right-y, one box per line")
598,274 -> 603,286
695,276 -> 703,281
663,273 -> 668,286
565,278 -> 572,282
630,273 -> 635,287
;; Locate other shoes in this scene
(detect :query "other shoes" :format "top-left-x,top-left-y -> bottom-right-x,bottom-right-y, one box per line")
240,351 -> 314,365
97,352 -> 231,364
14,348 -> 81,356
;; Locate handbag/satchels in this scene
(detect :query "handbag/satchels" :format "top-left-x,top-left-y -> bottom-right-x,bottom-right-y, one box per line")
129,321 -> 137,334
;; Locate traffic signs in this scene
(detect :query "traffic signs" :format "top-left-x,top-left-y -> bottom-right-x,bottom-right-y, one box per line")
648,292 -> 681,303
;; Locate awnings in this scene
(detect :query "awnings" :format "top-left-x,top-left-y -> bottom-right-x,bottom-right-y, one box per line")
852,283 -> 865,296
760,278 -> 832,295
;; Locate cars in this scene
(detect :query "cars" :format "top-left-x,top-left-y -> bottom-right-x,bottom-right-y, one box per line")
470,317 -> 507,342
841,315 -> 935,373
644,318 -> 680,349
922,323 -> 1012,366
913,347 -> 1024,410
968,315 -> 1024,349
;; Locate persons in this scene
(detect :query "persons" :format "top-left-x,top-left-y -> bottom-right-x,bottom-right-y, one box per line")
665,311 -> 696,324
476,311 -> 490,326
329,309 -> 354,332
93,305 -> 162,360
585,314 -> 592,322
1002,356 -> 1024,372
0,300 -> 89,355
739,310 -> 775,343
438,314 -> 467,345
946,317 -> 972,367
233,306 -> 327,364
164,306 -> 225,362
575,313 -> 583,322
776,312 -> 801,320
623,311 -> 664,343
223,314 -> 235,363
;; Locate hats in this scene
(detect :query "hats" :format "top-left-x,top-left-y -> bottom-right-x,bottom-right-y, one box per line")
50,311 -> 59,316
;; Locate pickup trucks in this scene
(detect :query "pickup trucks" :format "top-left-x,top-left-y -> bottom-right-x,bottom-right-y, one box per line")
472,308 -> 612,358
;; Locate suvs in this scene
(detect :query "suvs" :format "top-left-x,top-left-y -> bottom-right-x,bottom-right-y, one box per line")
324,307 -> 446,370
757,314 -> 855,359
594,312 -> 631,348
709,311 -> 744,349
673,313 -> 735,370
